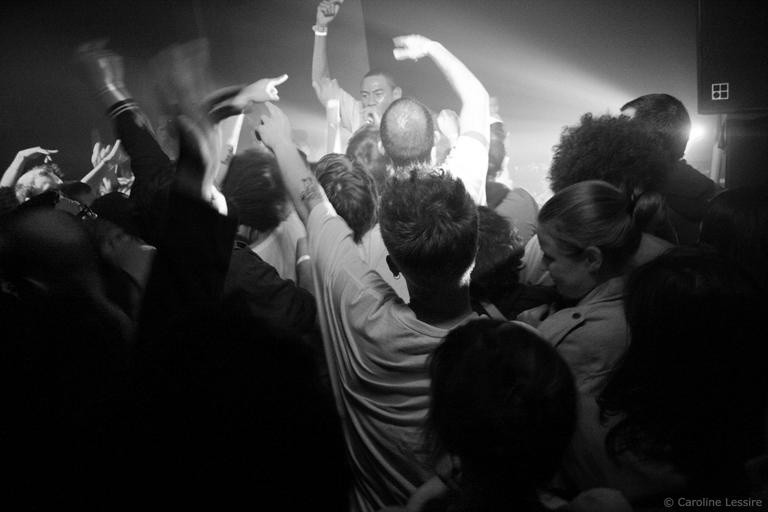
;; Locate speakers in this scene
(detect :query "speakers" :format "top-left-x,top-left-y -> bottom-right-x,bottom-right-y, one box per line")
696,0 -> 768,116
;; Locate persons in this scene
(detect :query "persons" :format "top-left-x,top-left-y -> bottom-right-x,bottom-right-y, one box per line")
1,1 -> 764,511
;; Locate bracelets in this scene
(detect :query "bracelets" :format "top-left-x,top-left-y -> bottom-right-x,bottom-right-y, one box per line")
312,25 -> 329,36
